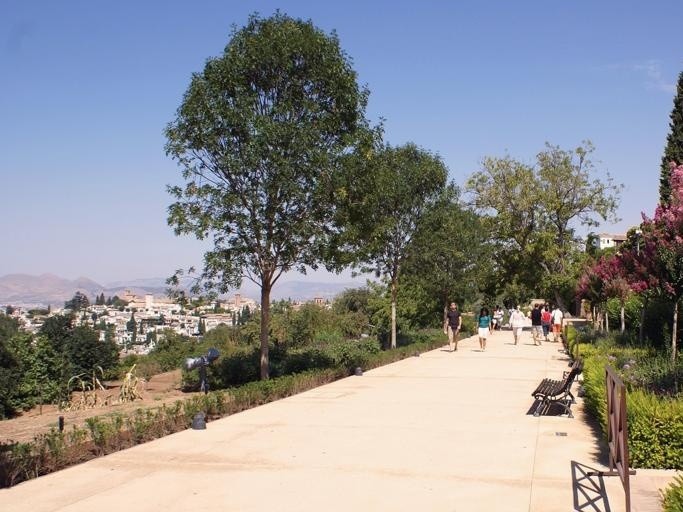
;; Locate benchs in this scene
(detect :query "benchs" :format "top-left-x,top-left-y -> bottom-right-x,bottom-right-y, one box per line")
532,360 -> 583,418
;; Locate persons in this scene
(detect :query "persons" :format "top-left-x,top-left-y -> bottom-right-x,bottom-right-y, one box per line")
444,302 -> 462,352
491,305 -> 573,346
477,307 -> 492,351
